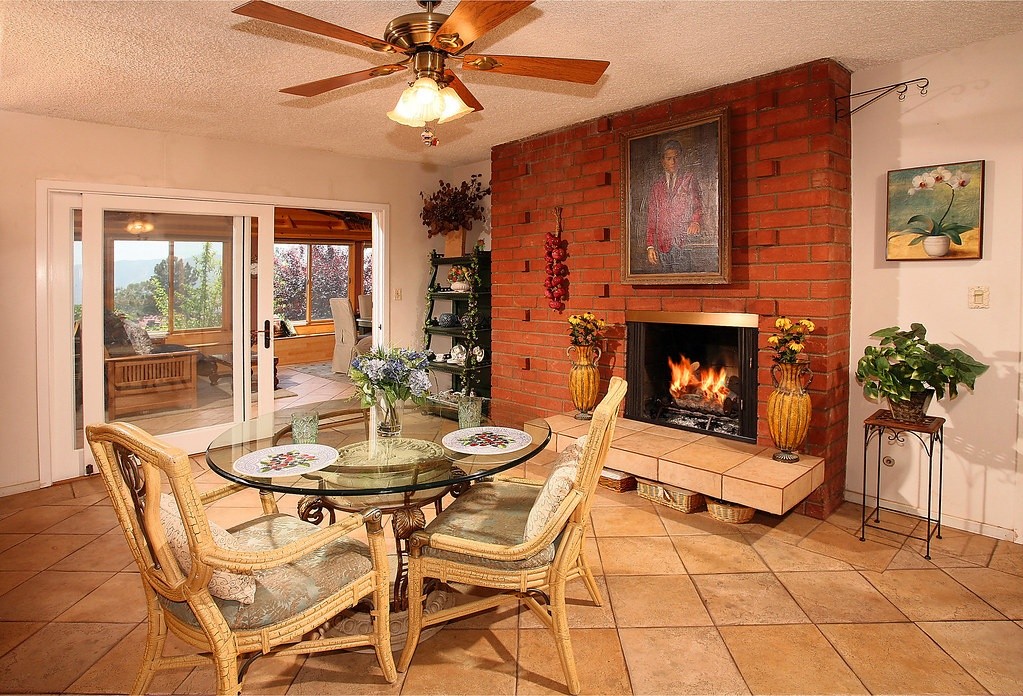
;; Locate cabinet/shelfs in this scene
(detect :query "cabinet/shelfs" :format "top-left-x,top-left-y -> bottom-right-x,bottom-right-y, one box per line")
419,248 -> 492,423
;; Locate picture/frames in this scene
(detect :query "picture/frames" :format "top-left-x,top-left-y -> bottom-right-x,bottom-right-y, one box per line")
619,103 -> 734,286
884,159 -> 985,262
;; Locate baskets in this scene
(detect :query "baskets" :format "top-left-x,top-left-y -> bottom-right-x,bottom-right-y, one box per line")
635,477 -> 705,514
704,495 -> 755,523
600,475 -> 637,492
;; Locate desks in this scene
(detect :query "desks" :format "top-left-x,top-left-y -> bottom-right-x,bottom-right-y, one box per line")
205,393 -> 552,653
356,318 -> 373,335
860,408 -> 946,560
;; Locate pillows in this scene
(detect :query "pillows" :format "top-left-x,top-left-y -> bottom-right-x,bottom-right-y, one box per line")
120,314 -> 156,354
104,309 -> 128,344
157,492 -> 257,604
524,433 -> 587,564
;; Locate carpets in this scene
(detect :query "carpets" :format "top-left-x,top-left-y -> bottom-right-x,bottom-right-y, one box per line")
76,375 -> 296,430
288,359 -> 351,384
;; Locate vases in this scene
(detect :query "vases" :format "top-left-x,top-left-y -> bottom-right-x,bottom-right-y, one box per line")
458,396 -> 482,429
439,313 -> 456,328
449,281 -> 470,292
565,345 -> 602,420
766,360 -> 814,463
444,226 -> 467,257
377,389 -> 405,437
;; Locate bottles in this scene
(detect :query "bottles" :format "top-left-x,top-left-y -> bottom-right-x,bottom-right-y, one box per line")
432,317 -> 438,326
437,283 -> 441,291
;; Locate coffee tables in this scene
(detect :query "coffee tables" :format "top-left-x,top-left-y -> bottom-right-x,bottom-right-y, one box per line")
209,351 -> 279,393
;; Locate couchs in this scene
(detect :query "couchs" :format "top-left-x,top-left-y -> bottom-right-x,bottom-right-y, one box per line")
104,337 -> 199,420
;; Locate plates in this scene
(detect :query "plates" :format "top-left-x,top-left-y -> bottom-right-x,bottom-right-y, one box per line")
432,359 -> 445,362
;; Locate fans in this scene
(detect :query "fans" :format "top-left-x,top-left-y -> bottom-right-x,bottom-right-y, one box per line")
232,0 -> 612,111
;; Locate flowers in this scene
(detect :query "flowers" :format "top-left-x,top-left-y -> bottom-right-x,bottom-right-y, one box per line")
446,265 -> 474,284
565,312 -> 605,346
448,238 -> 486,403
418,172 -> 491,239
347,345 -> 431,406
767,315 -> 816,360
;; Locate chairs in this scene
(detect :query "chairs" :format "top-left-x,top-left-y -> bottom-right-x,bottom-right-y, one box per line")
329,297 -> 372,372
396,374 -> 627,695
83,420 -> 400,696
358,295 -> 372,317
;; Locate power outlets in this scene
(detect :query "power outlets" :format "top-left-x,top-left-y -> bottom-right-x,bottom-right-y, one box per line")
395,289 -> 403,301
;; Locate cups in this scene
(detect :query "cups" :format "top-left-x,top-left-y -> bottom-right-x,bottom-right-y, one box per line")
434,354 -> 443,361
458,397 -> 481,428
443,353 -> 451,359
291,411 -> 318,444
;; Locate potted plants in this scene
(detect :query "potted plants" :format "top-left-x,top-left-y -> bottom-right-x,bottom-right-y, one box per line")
854,322 -> 990,422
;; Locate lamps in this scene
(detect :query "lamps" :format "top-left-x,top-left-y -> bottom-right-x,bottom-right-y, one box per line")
123,212 -> 158,239
384,50 -> 475,149
